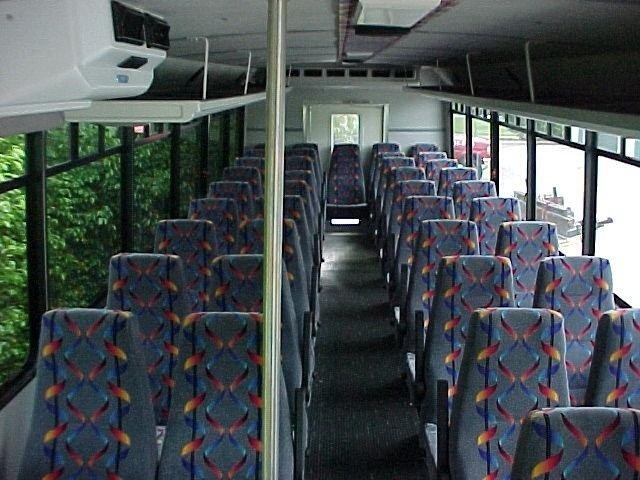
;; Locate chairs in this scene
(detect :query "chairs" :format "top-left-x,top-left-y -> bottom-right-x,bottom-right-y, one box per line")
17,143 -> 325,479
369,142 -> 640,478
326,141 -> 368,220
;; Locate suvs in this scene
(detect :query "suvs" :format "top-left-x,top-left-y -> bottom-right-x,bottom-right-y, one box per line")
453,133 -> 491,180
